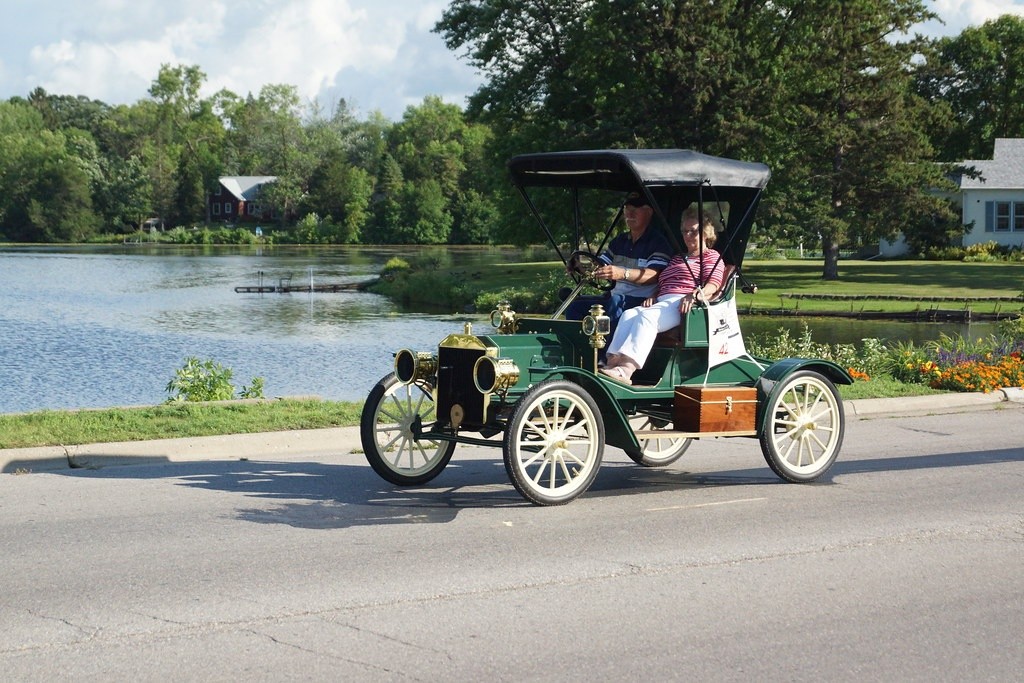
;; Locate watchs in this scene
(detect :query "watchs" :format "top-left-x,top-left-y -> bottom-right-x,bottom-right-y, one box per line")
621,266 -> 630,279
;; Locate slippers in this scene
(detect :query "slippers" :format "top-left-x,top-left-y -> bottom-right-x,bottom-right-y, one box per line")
598,365 -> 632,385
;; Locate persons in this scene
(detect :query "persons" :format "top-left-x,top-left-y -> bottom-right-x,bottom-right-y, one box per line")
595,204 -> 724,386
565,188 -> 677,367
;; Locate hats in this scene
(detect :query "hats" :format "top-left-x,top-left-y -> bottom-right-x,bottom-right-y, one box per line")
622,188 -> 651,207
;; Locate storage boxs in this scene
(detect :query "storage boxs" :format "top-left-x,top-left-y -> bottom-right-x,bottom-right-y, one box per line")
673,385 -> 757,431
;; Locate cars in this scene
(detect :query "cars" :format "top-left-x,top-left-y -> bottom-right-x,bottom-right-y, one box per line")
358,147 -> 860,510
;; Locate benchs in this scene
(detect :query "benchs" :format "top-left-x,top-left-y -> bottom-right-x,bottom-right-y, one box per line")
560,264 -> 735,346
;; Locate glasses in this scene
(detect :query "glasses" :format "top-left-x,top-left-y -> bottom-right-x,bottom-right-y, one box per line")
681,228 -> 699,235
